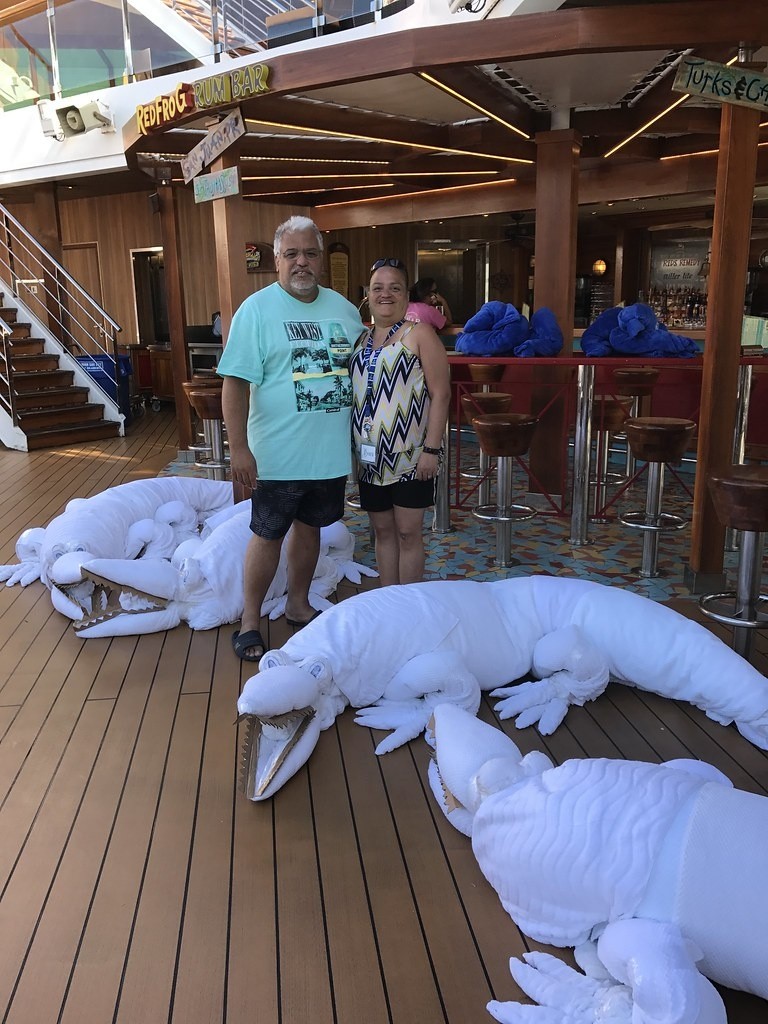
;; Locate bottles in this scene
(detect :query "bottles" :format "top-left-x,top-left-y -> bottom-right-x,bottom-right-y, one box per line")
638,284 -> 707,328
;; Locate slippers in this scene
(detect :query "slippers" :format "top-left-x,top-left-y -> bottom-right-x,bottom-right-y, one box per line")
286,609 -> 321,626
231,629 -> 265,662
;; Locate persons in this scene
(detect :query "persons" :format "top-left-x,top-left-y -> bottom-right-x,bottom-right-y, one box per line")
404,277 -> 454,333
216,214 -> 371,662
349,258 -> 453,589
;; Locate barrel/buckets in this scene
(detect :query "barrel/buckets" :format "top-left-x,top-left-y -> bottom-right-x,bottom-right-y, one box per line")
74,354 -> 133,426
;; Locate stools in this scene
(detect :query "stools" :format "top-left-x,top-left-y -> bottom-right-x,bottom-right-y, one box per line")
696,464 -> 768,662
469,412 -> 540,566
183,379 -> 225,479
193,372 -> 225,468
461,392 -> 514,510
590,395 -> 635,523
617,417 -> 697,578
469,362 -> 506,476
612,367 -> 658,485
190,387 -> 230,482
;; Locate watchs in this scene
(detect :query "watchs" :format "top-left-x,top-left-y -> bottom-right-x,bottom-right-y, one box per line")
422,444 -> 440,455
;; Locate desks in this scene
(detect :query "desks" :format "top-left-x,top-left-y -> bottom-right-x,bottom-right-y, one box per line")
146,340 -> 176,413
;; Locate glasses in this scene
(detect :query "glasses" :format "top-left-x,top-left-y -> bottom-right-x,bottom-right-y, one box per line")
431,288 -> 439,294
370,258 -> 409,273
280,248 -> 322,259
